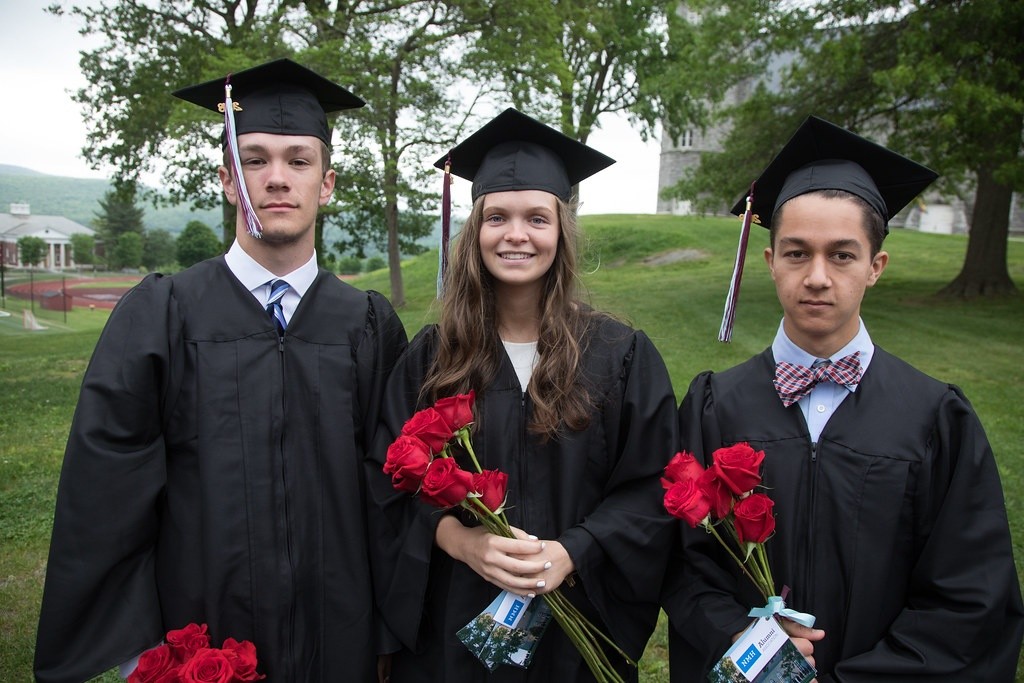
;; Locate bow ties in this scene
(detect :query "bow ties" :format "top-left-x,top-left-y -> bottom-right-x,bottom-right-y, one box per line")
773,351 -> 862,409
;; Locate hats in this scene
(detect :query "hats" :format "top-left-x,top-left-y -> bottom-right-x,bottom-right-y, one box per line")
433,107 -> 617,299
171,57 -> 365,239
717,113 -> 938,343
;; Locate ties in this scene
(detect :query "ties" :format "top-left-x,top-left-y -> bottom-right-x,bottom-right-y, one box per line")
262,279 -> 290,337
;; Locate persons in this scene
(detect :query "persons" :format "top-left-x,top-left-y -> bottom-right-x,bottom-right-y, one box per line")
662,113 -> 1023,682
366,107 -> 682,683
34,57 -> 412,683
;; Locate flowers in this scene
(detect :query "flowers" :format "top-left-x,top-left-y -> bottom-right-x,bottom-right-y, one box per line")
383,390 -> 638,683
127,623 -> 266,683
661,441 -> 783,628
